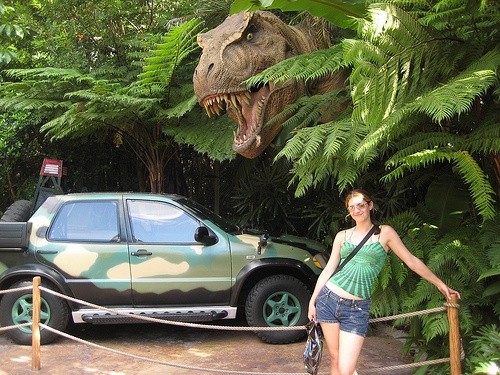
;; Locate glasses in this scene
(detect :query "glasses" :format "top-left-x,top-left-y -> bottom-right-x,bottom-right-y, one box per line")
347,202 -> 370,212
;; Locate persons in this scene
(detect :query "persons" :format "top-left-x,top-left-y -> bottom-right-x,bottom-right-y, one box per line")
308,191 -> 461,375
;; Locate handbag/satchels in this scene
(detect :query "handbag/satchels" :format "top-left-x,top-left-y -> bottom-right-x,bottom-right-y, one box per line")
303,320 -> 323,375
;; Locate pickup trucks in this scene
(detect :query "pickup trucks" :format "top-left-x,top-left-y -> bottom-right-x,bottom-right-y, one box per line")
0,191 -> 330,346
